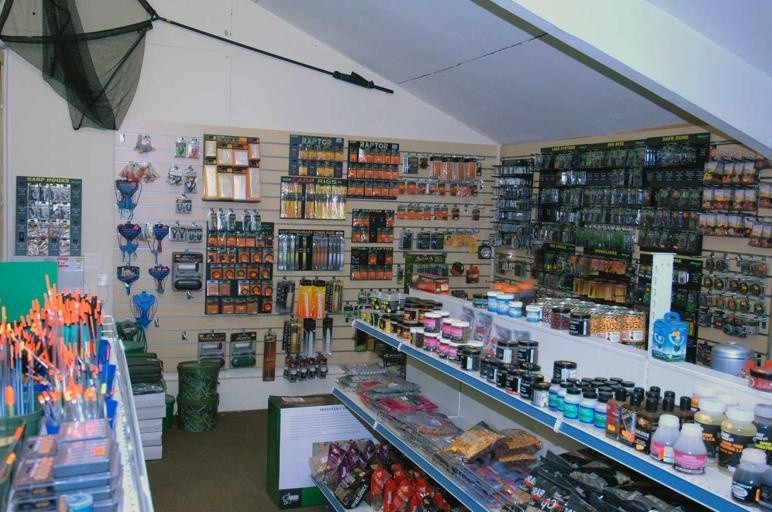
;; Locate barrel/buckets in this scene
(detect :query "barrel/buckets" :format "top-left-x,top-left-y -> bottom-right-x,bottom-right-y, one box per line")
177,359 -> 221,402
175,394 -> 220,434
163,395 -> 174,428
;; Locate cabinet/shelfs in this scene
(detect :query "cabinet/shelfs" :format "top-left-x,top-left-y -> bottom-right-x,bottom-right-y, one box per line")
0,312 -> 153,512
310,282 -> 772,512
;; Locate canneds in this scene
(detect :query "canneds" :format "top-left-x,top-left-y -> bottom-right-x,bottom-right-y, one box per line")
401,297 -> 646,408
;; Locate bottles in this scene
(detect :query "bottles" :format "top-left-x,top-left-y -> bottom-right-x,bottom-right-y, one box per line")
548,378 -> 772,512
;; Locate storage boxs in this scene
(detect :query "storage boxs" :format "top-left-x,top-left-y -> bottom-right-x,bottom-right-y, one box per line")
266,393 -> 390,510
